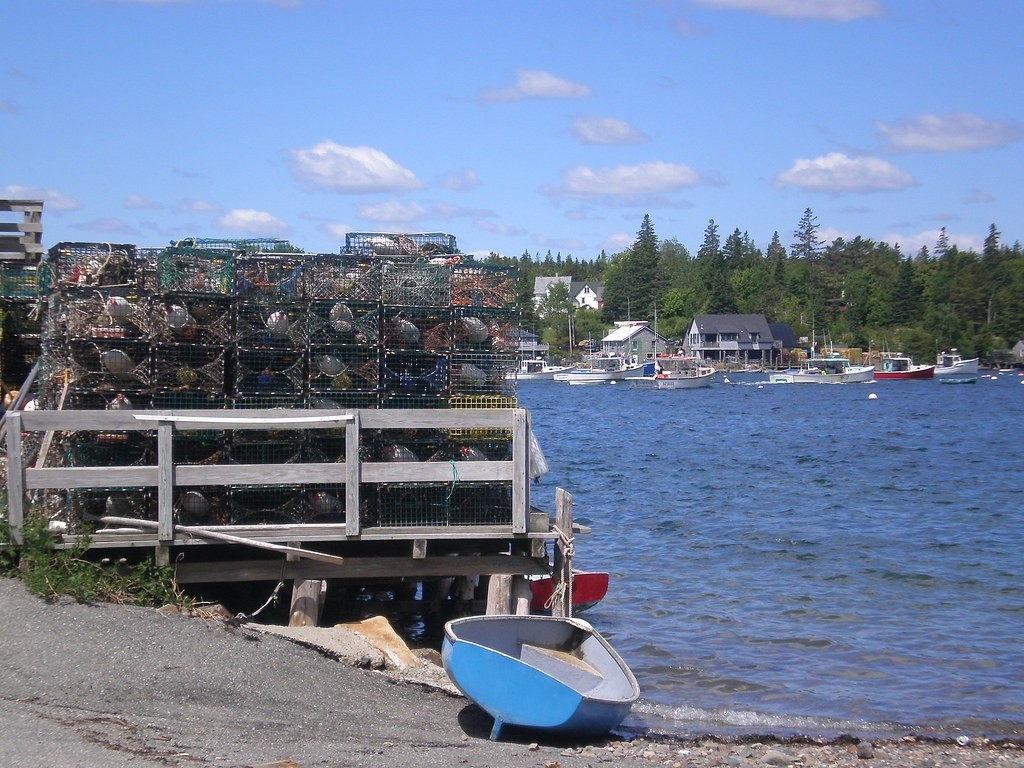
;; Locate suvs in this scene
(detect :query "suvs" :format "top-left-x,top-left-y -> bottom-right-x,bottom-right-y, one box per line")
578,341 -> 593,349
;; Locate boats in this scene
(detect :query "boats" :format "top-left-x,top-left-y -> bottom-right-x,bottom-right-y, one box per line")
501,553 -> 610,615
503,360 -> 574,380
874,358 -> 936,378
920,348 -> 979,374
939,377 -> 977,384
624,367 -> 716,389
769,357 -> 874,383
441,614 -> 640,741
569,380 -> 606,385
553,357 -> 644,380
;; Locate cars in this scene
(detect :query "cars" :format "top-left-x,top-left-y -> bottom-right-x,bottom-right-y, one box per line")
664,340 -> 677,346
